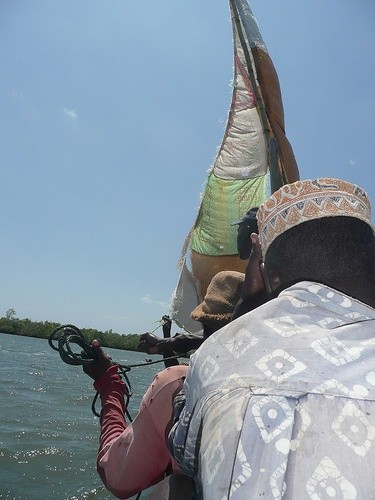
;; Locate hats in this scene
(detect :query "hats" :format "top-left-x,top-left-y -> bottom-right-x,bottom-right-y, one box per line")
256,178 -> 372,263
190,271 -> 245,331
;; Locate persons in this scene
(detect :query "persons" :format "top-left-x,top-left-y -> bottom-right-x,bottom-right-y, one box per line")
165,178 -> 375,500
82,271 -> 246,500
